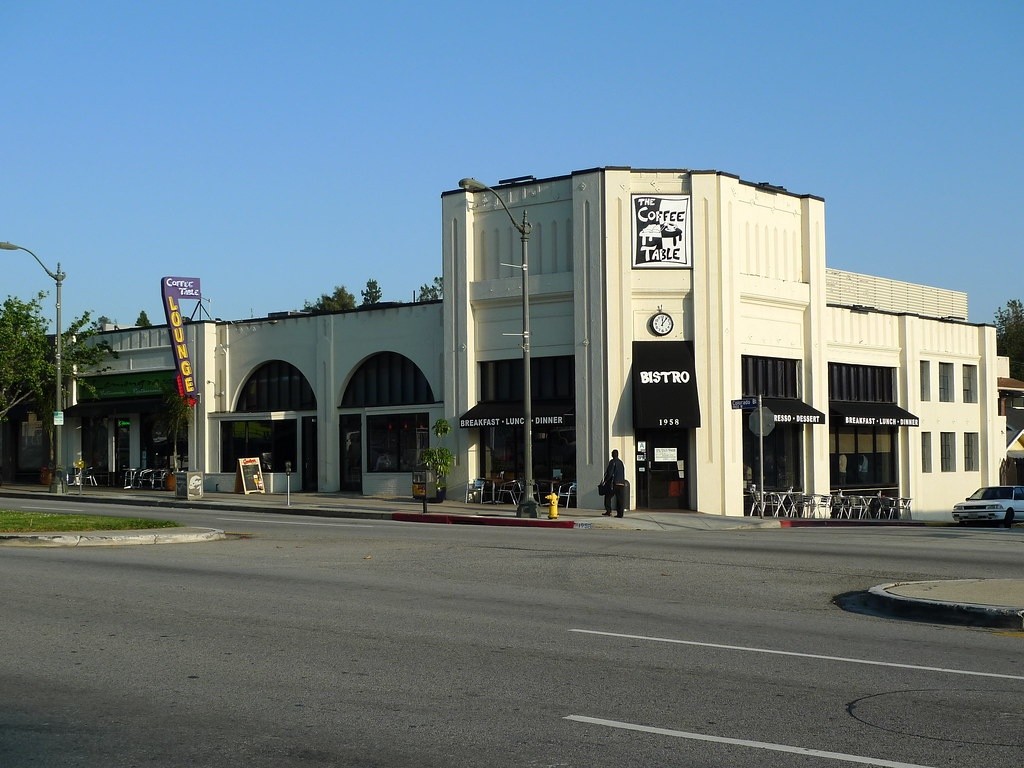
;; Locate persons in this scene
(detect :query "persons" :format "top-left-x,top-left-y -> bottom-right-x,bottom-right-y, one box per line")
602,450 -> 624,517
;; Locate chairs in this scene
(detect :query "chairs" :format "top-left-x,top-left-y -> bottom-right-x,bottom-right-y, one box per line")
466,482 -> 576,508
749,490 -> 913,522
66,466 -> 170,490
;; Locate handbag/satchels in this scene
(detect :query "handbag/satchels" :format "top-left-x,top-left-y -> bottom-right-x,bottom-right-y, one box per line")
598,473 -> 615,495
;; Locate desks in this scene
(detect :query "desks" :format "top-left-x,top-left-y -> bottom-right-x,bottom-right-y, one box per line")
538,479 -> 564,507
478,479 -> 505,505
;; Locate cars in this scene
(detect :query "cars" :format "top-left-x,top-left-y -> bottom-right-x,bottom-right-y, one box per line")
950,484 -> 1023,529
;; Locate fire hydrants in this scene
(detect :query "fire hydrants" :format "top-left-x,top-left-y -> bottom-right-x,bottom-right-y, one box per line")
545,493 -> 560,519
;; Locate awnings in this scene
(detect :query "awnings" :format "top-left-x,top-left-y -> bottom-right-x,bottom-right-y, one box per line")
829,400 -> 919,426
742,395 -> 825,424
632,341 -> 701,427
460,401 -> 575,428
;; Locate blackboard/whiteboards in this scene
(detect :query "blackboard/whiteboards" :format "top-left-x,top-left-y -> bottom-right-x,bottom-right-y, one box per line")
239,457 -> 266,495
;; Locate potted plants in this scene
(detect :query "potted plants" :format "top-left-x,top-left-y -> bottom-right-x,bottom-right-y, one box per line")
419,417 -> 454,505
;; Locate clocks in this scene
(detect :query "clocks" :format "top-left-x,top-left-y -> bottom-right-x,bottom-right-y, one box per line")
650,313 -> 674,336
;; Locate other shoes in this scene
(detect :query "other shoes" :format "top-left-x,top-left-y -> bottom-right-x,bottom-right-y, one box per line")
602,512 -> 610,516
614,514 -> 623,518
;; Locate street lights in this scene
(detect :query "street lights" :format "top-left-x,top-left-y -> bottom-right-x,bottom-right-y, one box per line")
457,175 -> 545,518
0,240 -> 69,494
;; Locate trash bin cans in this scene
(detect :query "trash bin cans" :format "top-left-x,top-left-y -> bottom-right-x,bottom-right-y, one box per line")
412,470 -> 437,513
173,470 -> 203,500
